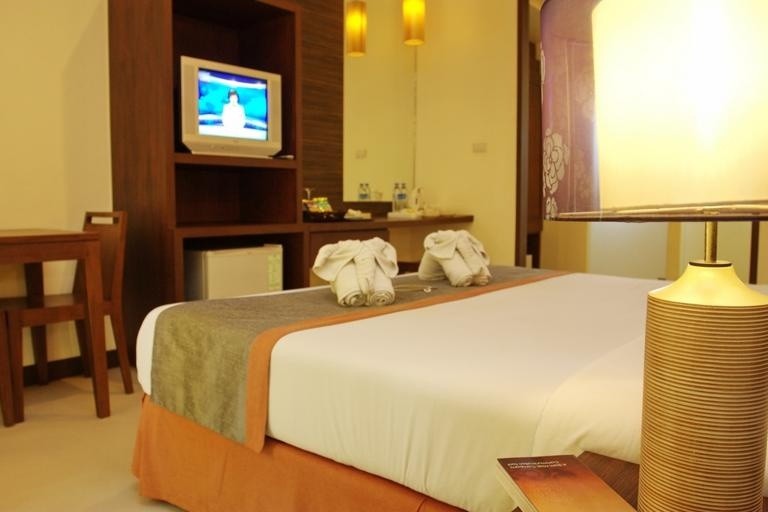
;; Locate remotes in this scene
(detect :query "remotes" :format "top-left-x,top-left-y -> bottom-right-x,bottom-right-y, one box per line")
275,154 -> 295,160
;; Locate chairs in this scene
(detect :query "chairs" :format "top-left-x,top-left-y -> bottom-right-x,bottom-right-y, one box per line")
1,210 -> 136,424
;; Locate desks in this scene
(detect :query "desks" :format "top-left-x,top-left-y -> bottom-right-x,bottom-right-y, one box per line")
305,215 -> 477,288
0,229 -> 111,427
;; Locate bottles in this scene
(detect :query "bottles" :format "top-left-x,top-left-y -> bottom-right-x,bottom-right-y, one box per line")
393,183 -> 408,209
358,182 -> 371,201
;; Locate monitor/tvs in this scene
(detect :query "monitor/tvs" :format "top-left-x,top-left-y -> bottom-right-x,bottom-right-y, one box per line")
180,54 -> 284,160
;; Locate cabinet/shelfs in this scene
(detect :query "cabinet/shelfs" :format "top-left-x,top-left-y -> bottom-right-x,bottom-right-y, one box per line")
107,0 -> 306,366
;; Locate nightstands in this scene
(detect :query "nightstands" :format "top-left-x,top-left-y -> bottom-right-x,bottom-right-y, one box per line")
510,448 -> 767,512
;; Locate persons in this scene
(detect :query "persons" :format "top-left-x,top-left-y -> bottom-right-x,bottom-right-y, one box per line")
221,87 -> 248,128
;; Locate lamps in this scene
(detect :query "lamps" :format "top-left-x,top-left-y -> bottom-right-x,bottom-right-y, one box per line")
345,2 -> 367,56
402,0 -> 426,47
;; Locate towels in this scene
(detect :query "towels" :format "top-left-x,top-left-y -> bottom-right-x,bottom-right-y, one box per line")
313,227 -> 495,308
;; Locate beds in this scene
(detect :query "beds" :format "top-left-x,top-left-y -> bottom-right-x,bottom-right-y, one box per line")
128,264 -> 768,512
